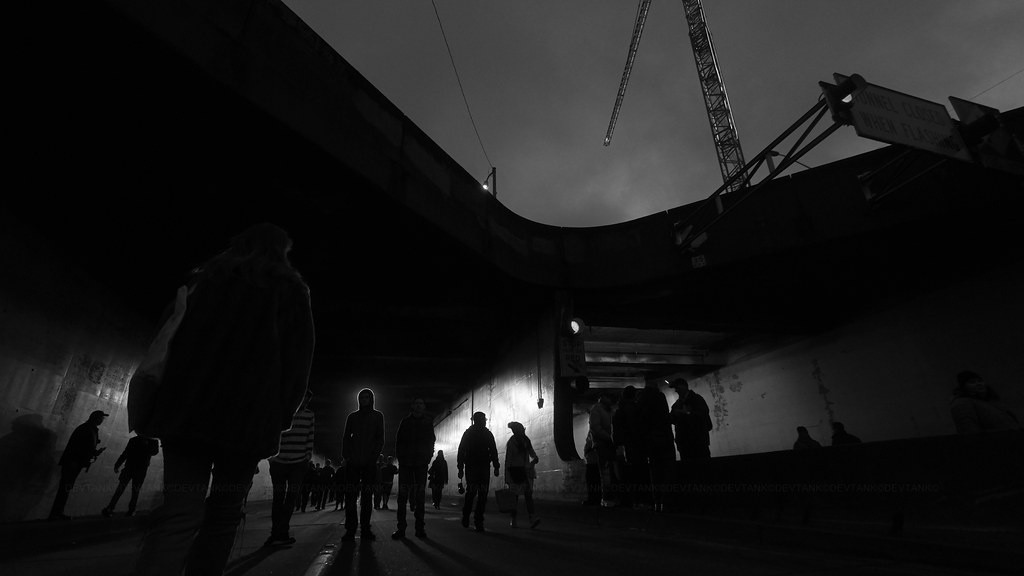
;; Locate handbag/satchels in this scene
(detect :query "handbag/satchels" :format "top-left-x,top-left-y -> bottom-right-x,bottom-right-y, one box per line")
128,281 -> 204,439
496,482 -> 516,514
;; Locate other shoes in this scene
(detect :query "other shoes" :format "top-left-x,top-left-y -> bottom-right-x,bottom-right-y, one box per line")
475,523 -> 485,533
124,510 -> 137,517
432,502 -> 435,506
462,513 -> 469,528
382,504 -> 388,510
509,520 -> 520,528
435,506 -> 440,510
415,529 -> 426,538
295,501 -> 346,513
410,505 -> 414,511
391,529 -> 405,538
361,529 -> 375,540
600,505 -> 609,512
47,513 -> 72,521
266,535 -> 297,546
101,508 -> 113,518
592,518 -> 600,528
374,504 -> 380,509
342,529 -> 356,541
529,516 -> 541,529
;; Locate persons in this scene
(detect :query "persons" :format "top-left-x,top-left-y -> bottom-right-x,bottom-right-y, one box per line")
391,397 -> 436,540
341,388 -> 385,539
296,453 -> 348,512
101,435 -> 159,517
669,378 -> 713,459
617,371 -> 677,513
429,450 -> 448,510
948,373 -> 1020,434
581,433 -> 602,505
265,389 -> 315,546
457,413 -> 500,532
505,422 -> 540,529
373,454 -> 399,510
48,411 -> 109,520
831,422 -> 861,444
793,426 -> 821,449
129,223 -> 316,576
591,392 -> 616,507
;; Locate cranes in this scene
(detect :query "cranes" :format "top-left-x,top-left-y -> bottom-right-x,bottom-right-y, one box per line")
602,0 -> 753,193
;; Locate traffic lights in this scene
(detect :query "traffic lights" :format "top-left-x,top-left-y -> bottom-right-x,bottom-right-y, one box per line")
562,316 -> 586,338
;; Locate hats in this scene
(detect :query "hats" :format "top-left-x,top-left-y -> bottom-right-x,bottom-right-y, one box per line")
957,370 -> 983,390
510,423 -> 525,434
91,411 -> 109,419
